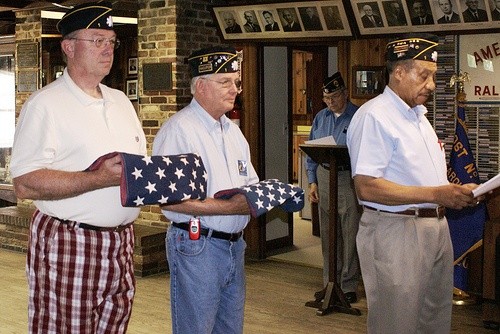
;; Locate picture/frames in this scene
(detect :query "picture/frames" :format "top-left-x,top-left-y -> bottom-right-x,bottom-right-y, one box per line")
346,0 -> 500,39
125,78 -> 138,101
126,55 -> 138,76
352,64 -> 387,100
208,0 -> 357,44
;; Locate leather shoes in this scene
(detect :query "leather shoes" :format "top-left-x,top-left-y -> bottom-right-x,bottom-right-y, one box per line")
315,290 -> 322,297
344,291 -> 357,303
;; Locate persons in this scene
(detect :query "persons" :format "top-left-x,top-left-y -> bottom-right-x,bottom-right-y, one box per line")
347,38 -> 493,333
218,0 -> 500,34
149,47 -> 260,333
5,4 -> 150,331
301,72 -> 361,312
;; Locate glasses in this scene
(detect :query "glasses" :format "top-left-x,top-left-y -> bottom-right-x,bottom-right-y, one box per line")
203,78 -> 243,89
66,37 -> 120,49
323,88 -> 345,102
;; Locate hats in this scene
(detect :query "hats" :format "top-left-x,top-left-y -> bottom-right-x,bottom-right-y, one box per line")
322,71 -> 345,94
55,2 -> 114,37
183,46 -> 238,80
385,35 -> 440,62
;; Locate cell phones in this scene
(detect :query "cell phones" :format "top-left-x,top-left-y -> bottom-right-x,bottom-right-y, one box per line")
189,218 -> 200,240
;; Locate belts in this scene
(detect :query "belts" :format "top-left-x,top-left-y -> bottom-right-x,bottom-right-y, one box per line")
171,219 -> 243,242
364,203 -> 446,219
51,215 -> 135,232
319,162 -> 351,172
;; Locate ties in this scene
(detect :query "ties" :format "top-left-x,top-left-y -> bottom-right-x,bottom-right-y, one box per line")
288,24 -> 291,28
446,16 -> 449,22
422,18 -> 425,25
369,16 -> 377,28
269,24 -> 273,31
231,28 -> 234,32
251,24 -> 256,32
474,13 -> 478,22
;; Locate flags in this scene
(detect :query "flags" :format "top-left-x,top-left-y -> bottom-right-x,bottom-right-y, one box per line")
81,150 -> 207,209
212,176 -> 307,218
446,107 -> 487,304
